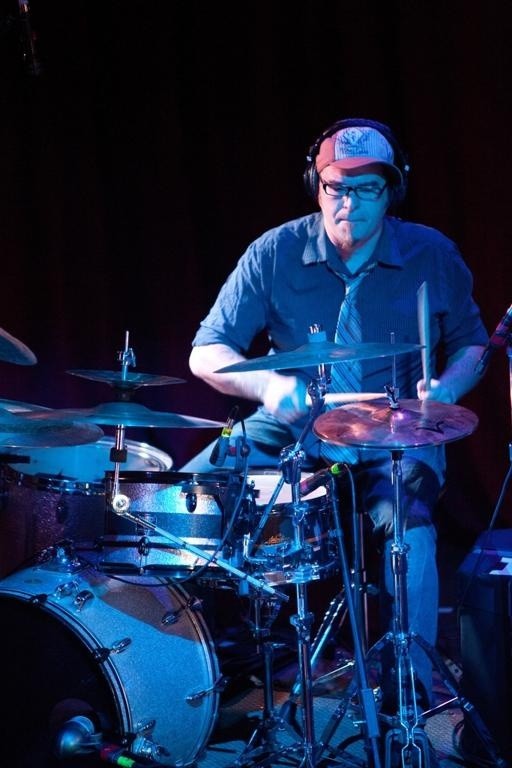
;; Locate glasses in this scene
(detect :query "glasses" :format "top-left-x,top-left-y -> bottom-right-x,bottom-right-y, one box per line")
320,177 -> 388,201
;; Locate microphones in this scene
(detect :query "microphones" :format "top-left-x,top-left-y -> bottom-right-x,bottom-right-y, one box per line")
472,304 -> 512,378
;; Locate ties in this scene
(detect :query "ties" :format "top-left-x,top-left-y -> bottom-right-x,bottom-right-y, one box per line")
321,265 -> 373,464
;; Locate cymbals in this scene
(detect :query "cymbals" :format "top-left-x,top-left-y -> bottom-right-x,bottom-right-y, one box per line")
0,327 -> 38,366
6,402 -> 227,430
68,366 -> 186,388
0,399 -> 104,447
312,396 -> 477,450
212,341 -> 427,375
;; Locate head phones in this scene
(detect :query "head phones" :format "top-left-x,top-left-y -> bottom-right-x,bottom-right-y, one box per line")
303,118 -> 414,203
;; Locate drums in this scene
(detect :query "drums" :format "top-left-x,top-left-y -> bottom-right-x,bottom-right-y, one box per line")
0,435 -> 174,581
219,470 -> 338,586
0,549 -> 223,767
99,470 -> 219,579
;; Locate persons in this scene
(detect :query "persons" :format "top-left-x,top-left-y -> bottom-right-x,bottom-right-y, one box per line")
178,116 -> 489,700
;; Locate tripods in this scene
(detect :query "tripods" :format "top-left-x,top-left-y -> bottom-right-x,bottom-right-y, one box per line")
230,456 -> 507,768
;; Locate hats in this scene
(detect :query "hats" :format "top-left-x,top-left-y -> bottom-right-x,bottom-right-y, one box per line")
316,127 -> 403,189
